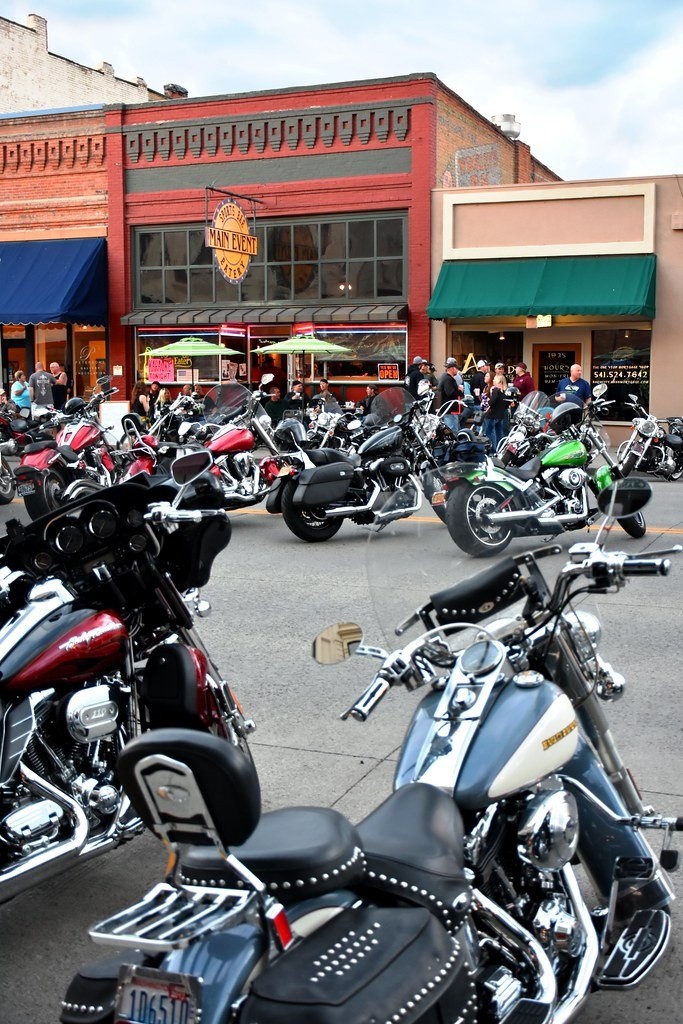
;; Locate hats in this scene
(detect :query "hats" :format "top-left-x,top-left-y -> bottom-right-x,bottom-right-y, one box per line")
427,362 -> 436,371
413,356 -> 422,365
495,363 -> 506,369
477,360 -> 492,369
446,358 -> 457,364
513,362 -> 527,370
447,363 -> 461,370
422,358 -> 431,366
0,389 -> 5,395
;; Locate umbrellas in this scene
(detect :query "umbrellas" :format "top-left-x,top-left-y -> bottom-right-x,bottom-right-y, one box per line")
251,332 -> 352,410
139,336 -> 243,392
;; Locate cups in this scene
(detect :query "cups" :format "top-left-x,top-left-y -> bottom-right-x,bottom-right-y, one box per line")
475,388 -> 480,396
296,393 -> 300,396
345,401 -> 349,408
560,392 -> 566,400
508,383 -> 513,388
459,385 -> 463,392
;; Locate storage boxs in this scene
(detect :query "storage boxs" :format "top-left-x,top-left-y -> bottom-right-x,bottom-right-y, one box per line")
292,461 -> 354,505
551,402 -> 583,435
57,951 -> 142,1024
242,906 -> 481,1024
266,477 -> 284,514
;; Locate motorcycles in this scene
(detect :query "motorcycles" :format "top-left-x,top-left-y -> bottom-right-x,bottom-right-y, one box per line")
57,542 -> 683,1024
1,372 -> 682,561
0,446 -> 260,918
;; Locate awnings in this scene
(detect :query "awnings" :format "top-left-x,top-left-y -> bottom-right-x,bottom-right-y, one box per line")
0,237 -> 109,327
426,253 -> 659,321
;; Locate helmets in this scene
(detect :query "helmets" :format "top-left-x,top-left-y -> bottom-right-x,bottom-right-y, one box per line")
549,402 -> 583,435
65,397 -> 86,414
505,387 -> 521,402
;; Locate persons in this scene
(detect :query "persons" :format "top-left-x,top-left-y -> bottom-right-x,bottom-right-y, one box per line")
438,357 -> 535,455
313,379 -> 339,407
555,364 -> 592,427
283,381 -> 310,409
264,356 -> 286,380
29,362 -> 67,419
264,387 -> 284,423
179,384 -> 216,418
0,388 -> 16,417
357,384 -> 390,436
132,381 -> 180,429
10,370 -> 31,419
404,356 -> 438,416
